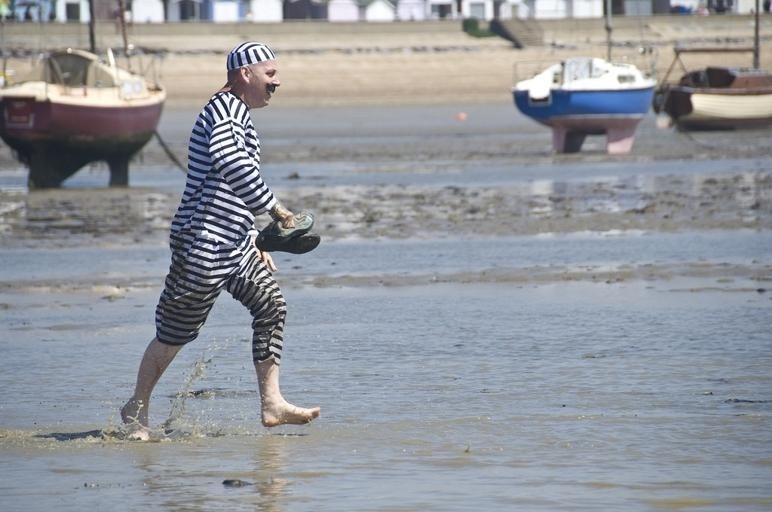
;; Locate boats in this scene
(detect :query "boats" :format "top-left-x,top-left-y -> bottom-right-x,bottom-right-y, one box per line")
663,64 -> 772,128
513,58 -> 657,156
0,45 -> 167,186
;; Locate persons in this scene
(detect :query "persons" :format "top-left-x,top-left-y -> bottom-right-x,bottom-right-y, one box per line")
119,37 -> 321,434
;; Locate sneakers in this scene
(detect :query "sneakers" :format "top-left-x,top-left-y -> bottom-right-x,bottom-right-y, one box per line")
255,210 -> 320,254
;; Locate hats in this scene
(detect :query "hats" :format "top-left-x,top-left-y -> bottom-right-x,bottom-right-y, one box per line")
227,40 -> 276,70
